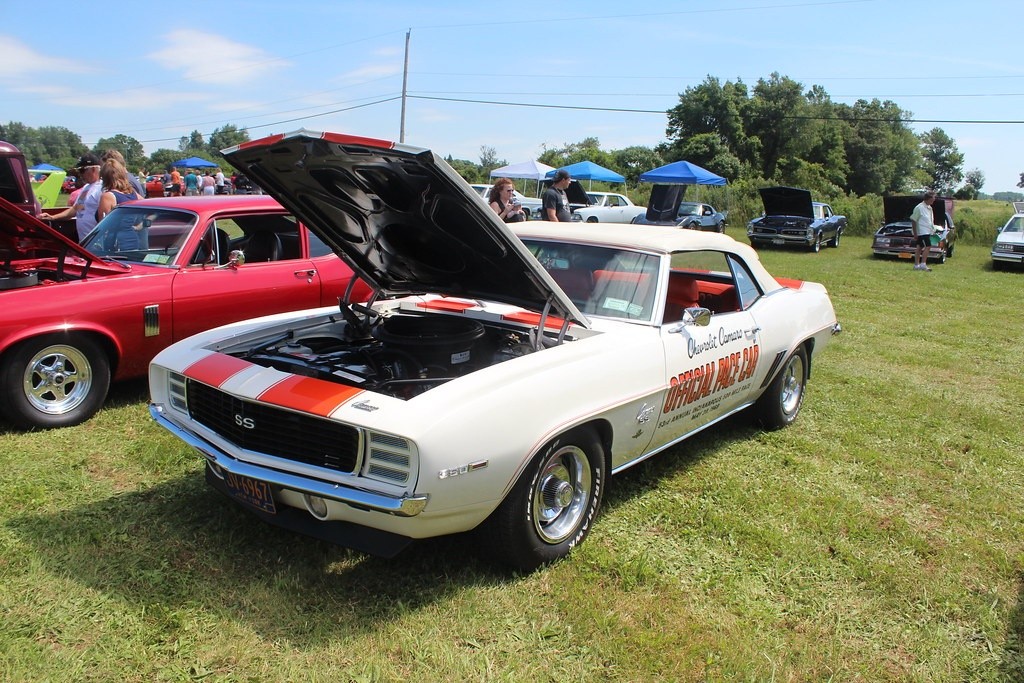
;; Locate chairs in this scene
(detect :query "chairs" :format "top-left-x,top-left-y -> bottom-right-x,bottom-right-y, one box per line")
547,268 -> 578,300
639,274 -> 701,325
194,229 -> 282,264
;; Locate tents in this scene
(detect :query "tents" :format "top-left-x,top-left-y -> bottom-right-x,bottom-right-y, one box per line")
171,156 -> 218,170
489,159 -> 556,198
540,161 -> 628,197
30,163 -> 62,171
639,160 -> 729,225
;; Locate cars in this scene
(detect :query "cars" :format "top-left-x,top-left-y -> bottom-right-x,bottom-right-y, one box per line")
635,185 -> 726,234
147,127 -> 842,575
34,175 -> 77,194
199,176 -> 234,194
991,213 -> 1024,272
539,178 -> 648,224
746,187 -> 847,253
468,183 -> 542,220
140,175 -> 188,196
0,194 -> 385,431
0,140 -> 80,247
871,195 -> 958,264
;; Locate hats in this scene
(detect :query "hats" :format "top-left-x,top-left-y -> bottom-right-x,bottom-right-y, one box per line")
74,154 -> 100,169
551,170 -> 570,181
186,168 -> 194,172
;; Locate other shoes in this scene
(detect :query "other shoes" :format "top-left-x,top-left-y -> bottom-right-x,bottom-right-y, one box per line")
913,266 -> 932,272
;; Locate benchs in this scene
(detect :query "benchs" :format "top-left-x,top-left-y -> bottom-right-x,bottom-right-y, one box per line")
591,270 -> 740,318
242,231 -> 300,262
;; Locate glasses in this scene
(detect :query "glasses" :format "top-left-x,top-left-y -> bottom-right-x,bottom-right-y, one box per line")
507,189 -> 513,192
80,168 -> 87,174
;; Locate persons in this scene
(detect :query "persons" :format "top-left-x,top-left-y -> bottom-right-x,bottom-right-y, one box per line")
29,150 -> 262,253
910,192 -> 935,272
540,170 -> 572,222
488,178 -> 526,222
